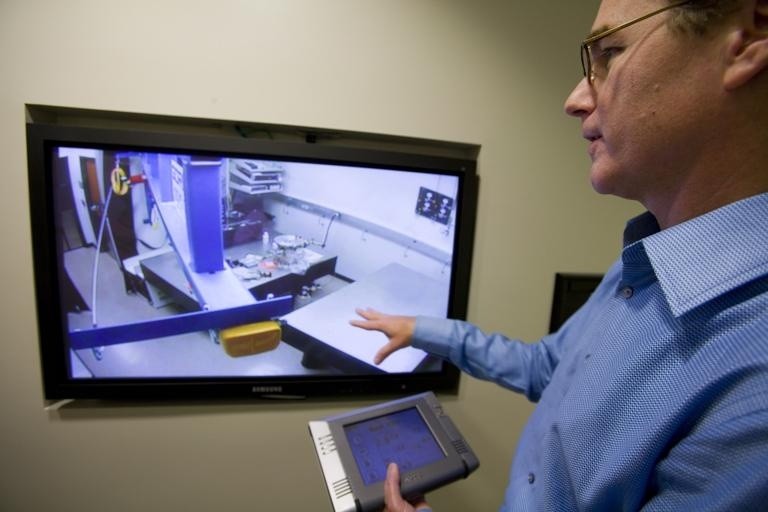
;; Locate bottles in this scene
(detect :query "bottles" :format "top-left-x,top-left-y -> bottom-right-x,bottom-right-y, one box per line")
262,232 -> 269,251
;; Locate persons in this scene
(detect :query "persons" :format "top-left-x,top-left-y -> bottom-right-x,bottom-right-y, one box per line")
349,1 -> 768,510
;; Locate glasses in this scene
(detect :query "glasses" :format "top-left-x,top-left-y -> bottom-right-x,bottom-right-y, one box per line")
580,0 -> 686,86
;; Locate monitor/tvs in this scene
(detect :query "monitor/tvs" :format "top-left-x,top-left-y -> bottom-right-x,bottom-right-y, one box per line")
24,102 -> 482,406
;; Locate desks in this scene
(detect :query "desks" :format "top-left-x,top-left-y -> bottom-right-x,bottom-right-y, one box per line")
137,232 -> 341,321
277,261 -> 450,374
